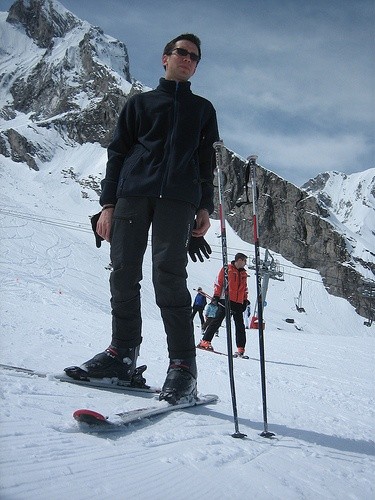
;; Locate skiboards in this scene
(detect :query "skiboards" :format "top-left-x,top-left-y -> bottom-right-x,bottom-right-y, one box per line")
0,364 -> 220,435
196,345 -> 250,360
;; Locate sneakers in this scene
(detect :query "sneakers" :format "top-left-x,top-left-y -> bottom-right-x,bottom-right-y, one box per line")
161,358 -> 196,395
200,340 -> 213,350
238,347 -> 244,353
82,345 -> 137,377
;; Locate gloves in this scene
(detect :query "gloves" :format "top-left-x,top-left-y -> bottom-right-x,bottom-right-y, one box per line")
90,213 -> 105,248
212,296 -> 220,305
242,300 -> 249,311
188,219 -> 212,262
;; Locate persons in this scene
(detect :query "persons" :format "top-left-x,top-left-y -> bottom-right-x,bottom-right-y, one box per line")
202,298 -> 219,337
198,253 -> 248,356
84,33 -> 217,402
243,307 -> 250,328
192,287 -> 207,324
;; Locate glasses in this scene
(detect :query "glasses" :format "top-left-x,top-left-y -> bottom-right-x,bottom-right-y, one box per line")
172,47 -> 200,62
